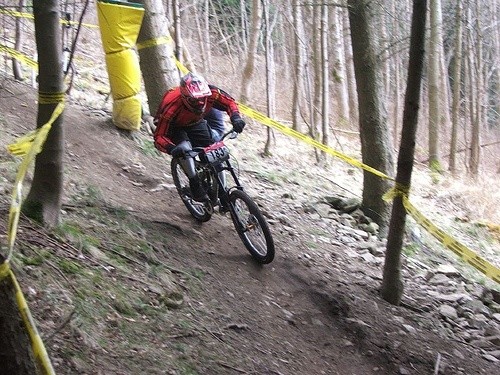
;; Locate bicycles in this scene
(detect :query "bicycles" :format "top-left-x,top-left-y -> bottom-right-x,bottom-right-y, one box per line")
170,126 -> 275,264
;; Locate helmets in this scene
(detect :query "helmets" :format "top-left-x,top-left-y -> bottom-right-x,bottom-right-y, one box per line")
180,72 -> 211,114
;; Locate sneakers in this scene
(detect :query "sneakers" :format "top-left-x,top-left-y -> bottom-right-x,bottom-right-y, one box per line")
189,184 -> 210,201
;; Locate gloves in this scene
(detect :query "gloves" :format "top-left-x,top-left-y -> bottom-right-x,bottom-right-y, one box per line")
231,114 -> 246,133
171,142 -> 192,157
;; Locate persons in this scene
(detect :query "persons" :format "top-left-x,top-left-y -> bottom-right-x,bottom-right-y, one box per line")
151,72 -> 245,214
